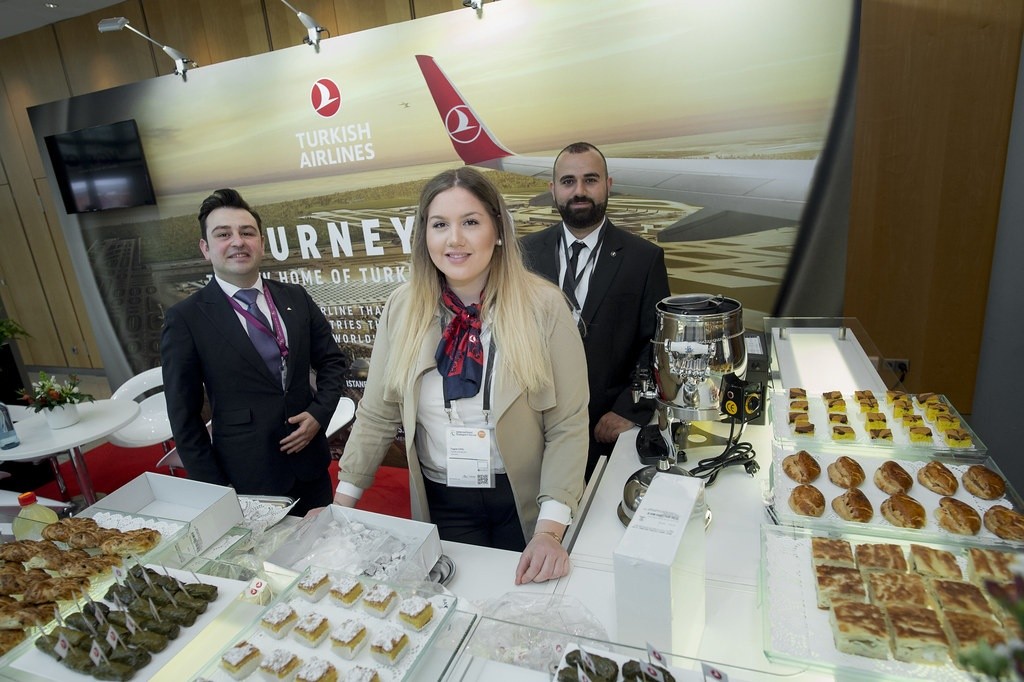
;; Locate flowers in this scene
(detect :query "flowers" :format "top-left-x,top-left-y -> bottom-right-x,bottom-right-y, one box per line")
15,369 -> 95,413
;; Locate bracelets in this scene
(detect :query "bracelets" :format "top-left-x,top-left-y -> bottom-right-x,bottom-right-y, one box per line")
532,530 -> 562,545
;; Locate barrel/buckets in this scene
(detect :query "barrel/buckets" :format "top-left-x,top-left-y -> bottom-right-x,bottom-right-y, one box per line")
653,294 -> 749,410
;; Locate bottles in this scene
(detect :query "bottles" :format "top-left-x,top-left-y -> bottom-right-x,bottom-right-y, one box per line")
0,402 -> 20,450
12,492 -> 59,543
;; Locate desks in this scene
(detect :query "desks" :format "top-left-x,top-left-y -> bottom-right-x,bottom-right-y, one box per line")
0,398 -> 140,503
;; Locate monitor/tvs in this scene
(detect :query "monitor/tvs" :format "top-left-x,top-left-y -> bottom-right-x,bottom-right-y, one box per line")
44,119 -> 157,215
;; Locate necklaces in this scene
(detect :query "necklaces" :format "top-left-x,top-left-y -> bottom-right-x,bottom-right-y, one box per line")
560,214 -> 608,311
437,292 -> 497,411
221,281 -> 289,358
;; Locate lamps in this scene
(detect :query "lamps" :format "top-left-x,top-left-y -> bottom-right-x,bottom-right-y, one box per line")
97,17 -> 199,77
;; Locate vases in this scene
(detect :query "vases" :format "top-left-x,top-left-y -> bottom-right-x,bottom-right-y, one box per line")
42,396 -> 80,429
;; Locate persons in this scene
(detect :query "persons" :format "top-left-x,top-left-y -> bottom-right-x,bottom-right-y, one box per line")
302,167 -> 590,585
515,142 -> 672,487
161,189 -> 347,518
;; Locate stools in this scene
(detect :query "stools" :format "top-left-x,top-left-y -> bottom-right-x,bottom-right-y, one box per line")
159,414 -> 213,470
104,364 -> 205,478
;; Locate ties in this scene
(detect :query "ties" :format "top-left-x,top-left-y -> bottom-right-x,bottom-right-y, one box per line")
562,242 -> 587,312
233,288 -> 282,386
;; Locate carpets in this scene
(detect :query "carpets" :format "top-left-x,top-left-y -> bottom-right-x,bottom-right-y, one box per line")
26,438 -> 412,528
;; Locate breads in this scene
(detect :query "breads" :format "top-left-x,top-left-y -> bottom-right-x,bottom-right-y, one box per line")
558,651 -> 677,682
0,515 -> 434,682
782,450 -> 1024,542
787,388 -> 972,449
812,537 -> 1024,671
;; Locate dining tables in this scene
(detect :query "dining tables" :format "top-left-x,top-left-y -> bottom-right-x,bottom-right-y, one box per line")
0,323 -> 1024,682
